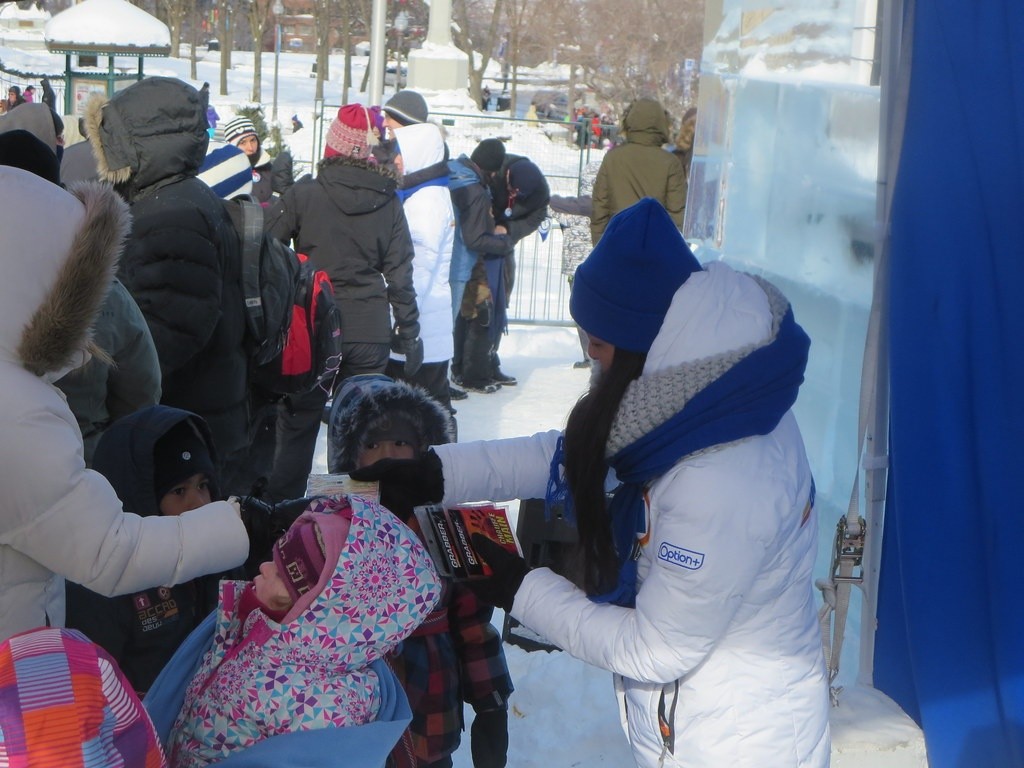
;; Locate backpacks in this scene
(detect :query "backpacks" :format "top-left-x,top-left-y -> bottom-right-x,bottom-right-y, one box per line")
220,194 -> 304,393
277,254 -> 343,396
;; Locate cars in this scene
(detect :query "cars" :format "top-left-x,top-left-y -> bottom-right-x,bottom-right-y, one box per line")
532,90 -> 577,120
384,65 -> 406,88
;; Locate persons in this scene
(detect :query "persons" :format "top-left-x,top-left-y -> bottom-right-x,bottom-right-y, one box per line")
591,98 -> 688,247
1,626 -> 166,768
53,271 -> 163,470
164,492 -> 441,768
463,197 -> 833,768
327,372 -> 516,768
672,106 -> 701,175
66,406 -> 211,696
0,162 -> 326,638
2,77 -> 696,538
84,75 -> 252,501
0,101 -> 64,189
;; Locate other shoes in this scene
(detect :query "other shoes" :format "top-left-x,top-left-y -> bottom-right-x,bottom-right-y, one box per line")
574,360 -> 592,368
450,367 -> 517,394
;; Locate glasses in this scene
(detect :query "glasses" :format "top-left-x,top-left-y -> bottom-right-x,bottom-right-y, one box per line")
57,135 -> 64,143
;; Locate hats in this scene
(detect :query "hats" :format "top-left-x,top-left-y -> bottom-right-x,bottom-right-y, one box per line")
292,114 -> 298,121
569,198 -> 703,353
471,138 -> 505,170
384,90 -> 429,126
157,419 -> 215,506
510,160 -> 542,200
194,142 -> 252,199
224,115 -> 261,148
273,522 -> 326,603
324,103 -> 379,160
364,413 -> 419,456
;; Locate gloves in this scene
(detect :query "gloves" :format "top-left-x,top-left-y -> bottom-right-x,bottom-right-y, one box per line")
348,457 -> 437,525
400,337 -> 424,378
472,532 -> 532,613
239,495 -> 323,554
470,703 -> 509,768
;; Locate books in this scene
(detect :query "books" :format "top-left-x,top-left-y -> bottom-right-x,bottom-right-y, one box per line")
412,495 -> 546,580
